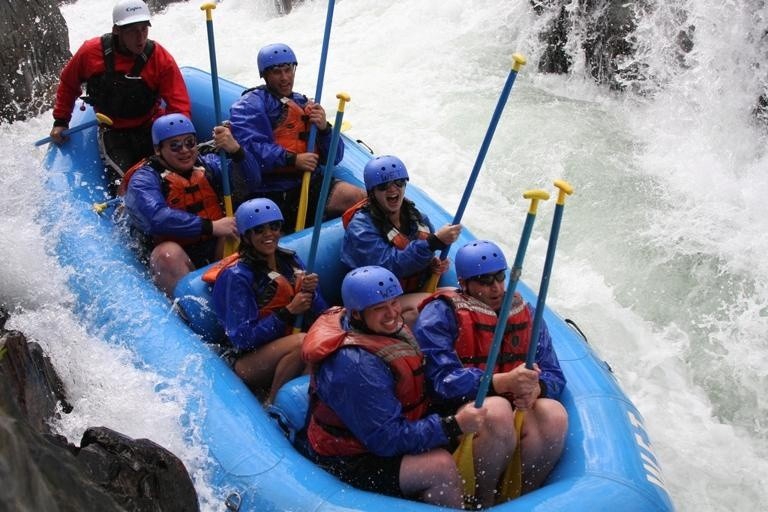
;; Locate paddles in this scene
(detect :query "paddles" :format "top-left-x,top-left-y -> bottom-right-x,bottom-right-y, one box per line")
451,190 -> 551,511
420,53 -> 527,296
199,4 -> 240,258
496,181 -> 574,503
296,0 -> 336,231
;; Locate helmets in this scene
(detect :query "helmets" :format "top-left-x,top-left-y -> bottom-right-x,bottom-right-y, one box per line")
112,0 -> 150,29
235,198 -> 283,235
455,241 -> 507,280
340,266 -> 402,311
257,43 -> 297,77
364,155 -> 408,190
151,113 -> 196,144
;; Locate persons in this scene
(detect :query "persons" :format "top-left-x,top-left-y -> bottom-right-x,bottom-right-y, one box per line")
47,0 -> 191,193
126,114 -> 265,297
227,42 -> 362,235
209,196 -> 327,408
339,154 -> 462,292
304,264 -> 488,509
411,237 -> 570,509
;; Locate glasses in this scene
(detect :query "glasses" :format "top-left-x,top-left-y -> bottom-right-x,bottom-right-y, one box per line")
253,221 -> 279,233
170,136 -> 195,151
376,178 -> 405,191
471,270 -> 505,285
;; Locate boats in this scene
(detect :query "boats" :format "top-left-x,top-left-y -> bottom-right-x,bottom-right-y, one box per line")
28,64 -> 677,511
